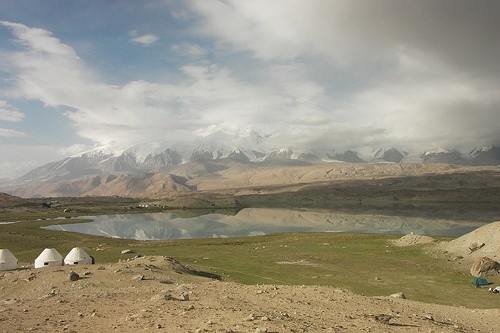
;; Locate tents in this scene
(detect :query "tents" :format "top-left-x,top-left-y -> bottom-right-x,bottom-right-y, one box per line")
35,247 -> 63,269
64,247 -> 93,265
0,249 -> 19,271
